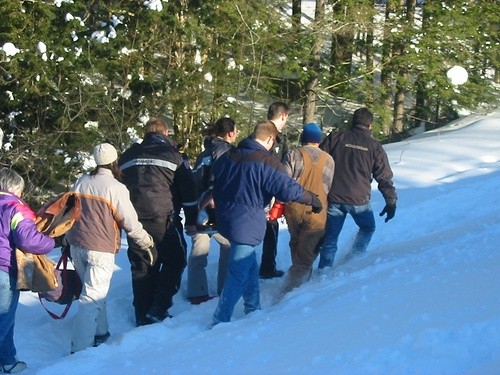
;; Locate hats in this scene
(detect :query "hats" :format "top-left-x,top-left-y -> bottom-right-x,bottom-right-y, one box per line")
300,123 -> 322,143
93,143 -> 118,165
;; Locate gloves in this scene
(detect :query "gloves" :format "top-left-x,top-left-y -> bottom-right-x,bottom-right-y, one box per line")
379,204 -> 396,223
269,202 -> 287,221
143,235 -> 157,266
312,197 -> 323,214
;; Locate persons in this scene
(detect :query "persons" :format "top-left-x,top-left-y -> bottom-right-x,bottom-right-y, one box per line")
248,102 -> 289,280
186,118 -> 238,304
318,108 -> 398,269
268,123 -> 335,305
62,143 -> 157,355
209,120 -> 323,332
0,167 -> 55,373
118,119 -> 198,327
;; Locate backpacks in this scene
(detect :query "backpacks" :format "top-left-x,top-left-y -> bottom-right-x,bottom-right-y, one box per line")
190,143 -> 228,233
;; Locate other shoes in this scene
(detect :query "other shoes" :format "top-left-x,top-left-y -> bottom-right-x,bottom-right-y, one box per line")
0,361 -> 27,373
259,270 -> 284,279
146,306 -> 174,323
191,296 -> 213,304
90,331 -> 111,347
136,318 -> 153,327
210,320 -> 219,328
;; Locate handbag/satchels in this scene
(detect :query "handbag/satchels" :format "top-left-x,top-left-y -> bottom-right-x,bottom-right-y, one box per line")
38,254 -> 82,319
